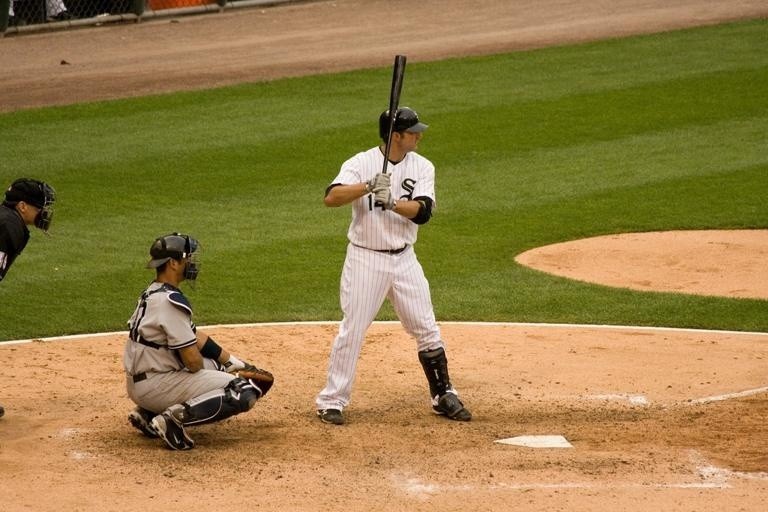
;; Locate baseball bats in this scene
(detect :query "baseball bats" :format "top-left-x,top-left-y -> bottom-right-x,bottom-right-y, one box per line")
373,54 -> 406,207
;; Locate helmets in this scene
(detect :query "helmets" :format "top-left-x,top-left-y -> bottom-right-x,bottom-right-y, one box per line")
4,178 -> 57,233
380,107 -> 429,138
145,231 -> 202,291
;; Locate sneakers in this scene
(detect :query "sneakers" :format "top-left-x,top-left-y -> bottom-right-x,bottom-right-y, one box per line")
432,392 -> 472,421
317,409 -> 344,425
149,410 -> 195,450
46,10 -> 76,21
7,16 -> 26,27
128,406 -> 160,438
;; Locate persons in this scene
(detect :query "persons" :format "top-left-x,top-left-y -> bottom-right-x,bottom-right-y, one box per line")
123,233 -> 274,450
1,176 -> 57,420
314,106 -> 471,424
7,1 -> 79,26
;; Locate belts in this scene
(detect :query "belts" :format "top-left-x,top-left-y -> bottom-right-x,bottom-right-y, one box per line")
133,371 -> 146,383
374,242 -> 407,254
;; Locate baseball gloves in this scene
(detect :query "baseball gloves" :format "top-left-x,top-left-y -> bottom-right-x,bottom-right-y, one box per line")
223,360 -> 274,399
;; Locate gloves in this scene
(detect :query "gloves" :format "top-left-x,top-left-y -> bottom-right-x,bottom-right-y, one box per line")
366,172 -> 396,210
